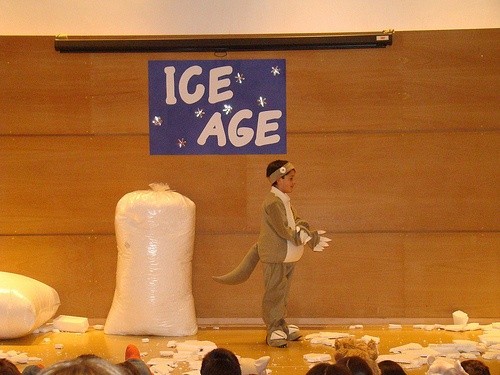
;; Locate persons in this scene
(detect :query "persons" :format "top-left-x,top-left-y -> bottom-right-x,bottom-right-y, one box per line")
335,356 -> 373,375
378,360 -> 406,375
0,358 -> 43,375
305,363 -> 351,375
460,360 -> 489,375
212,159 -> 332,348
37,344 -> 151,375
200,349 -> 241,375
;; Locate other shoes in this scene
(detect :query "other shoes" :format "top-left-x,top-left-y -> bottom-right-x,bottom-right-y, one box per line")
267,330 -> 288,347
288,324 -> 302,340
